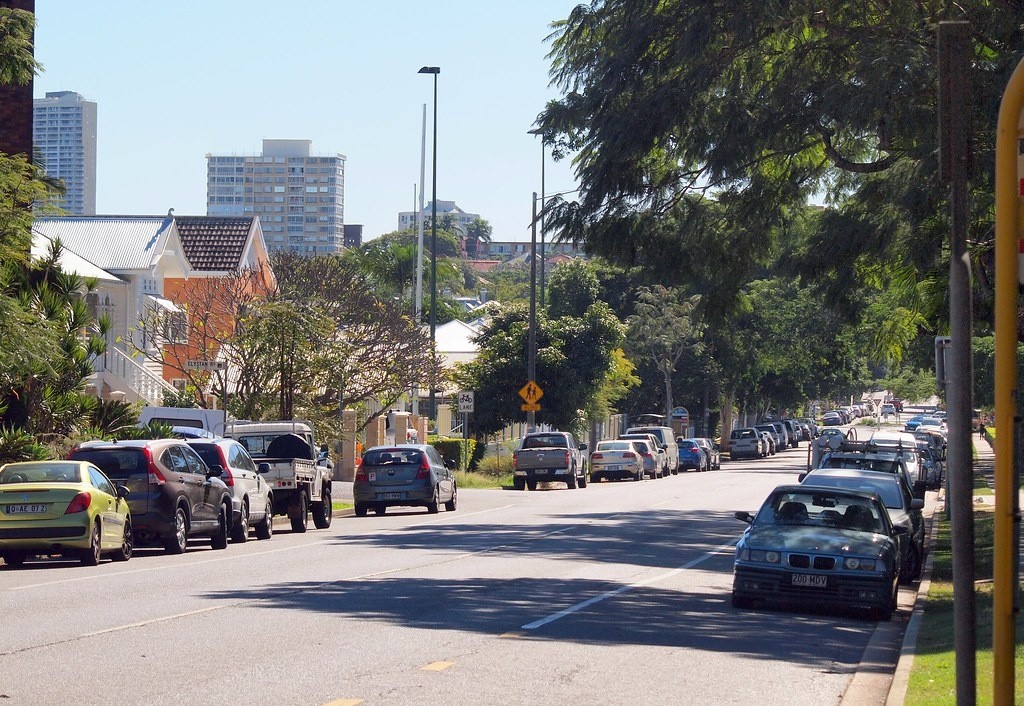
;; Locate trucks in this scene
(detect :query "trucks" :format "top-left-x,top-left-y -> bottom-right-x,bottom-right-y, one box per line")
213,420 -> 333,532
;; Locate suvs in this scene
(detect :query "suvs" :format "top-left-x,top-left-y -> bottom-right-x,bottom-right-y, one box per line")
64,438 -> 232,553
183,439 -> 273,541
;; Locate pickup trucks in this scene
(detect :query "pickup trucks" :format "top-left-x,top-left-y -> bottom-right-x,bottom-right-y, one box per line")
512,432 -> 587,490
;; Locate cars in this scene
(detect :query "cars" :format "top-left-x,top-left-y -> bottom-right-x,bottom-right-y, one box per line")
798,427 -> 943,579
821,402 -> 872,426
728,428 -> 763,460
589,440 -> 645,482
615,426 -> 720,480
880,404 -> 896,416
0,460 -> 134,566
731,484 -> 908,620
753,418 -> 818,457
353,443 -> 457,516
915,410 -> 949,461
904,415 -> 925,431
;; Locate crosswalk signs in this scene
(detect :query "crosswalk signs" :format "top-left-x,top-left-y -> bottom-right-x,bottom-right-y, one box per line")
519,380 -> 545,404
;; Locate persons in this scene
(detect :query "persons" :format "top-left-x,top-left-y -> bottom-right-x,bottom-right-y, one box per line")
978,420 -> 985,440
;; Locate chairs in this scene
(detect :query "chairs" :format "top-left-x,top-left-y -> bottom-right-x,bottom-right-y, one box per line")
100,456 -> 120,475
842,505 -> 875,529
775,502 -> 807,523
380,454 -> 392,463
8,473 -> 28,483
